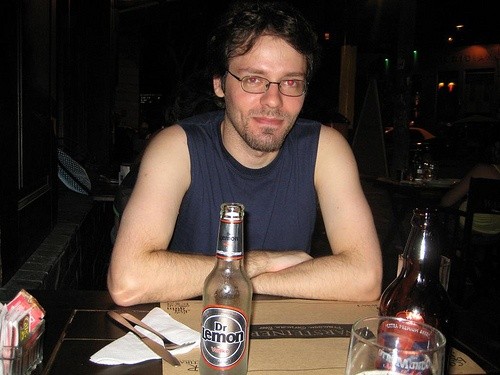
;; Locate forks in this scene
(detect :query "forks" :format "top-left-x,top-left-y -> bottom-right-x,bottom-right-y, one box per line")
116,309 -> 195,351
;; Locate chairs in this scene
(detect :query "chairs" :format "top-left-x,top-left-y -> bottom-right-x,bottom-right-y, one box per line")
432,177 -> 500,292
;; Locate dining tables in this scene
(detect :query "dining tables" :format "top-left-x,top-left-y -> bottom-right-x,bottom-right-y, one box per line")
43,306 -> 500,375
376,174 -> 461,247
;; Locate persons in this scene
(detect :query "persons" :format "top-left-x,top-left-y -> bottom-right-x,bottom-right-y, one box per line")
423,134 -> 500,245
103,0 -> 383,308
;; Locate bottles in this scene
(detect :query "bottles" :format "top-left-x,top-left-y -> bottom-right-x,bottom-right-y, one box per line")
379,207 -> 452,375
199,202 -> 252,375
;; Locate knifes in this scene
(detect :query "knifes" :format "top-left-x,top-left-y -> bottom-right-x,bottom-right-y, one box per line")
108,310 -> 180,368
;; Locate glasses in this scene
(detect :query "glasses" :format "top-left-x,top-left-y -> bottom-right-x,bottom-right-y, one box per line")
227,66 -> 309,98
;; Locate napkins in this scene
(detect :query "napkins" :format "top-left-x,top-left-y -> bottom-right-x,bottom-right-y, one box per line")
90,307 -> 200,364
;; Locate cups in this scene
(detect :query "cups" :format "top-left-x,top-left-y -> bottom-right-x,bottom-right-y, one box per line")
346,315 -> 447,375
